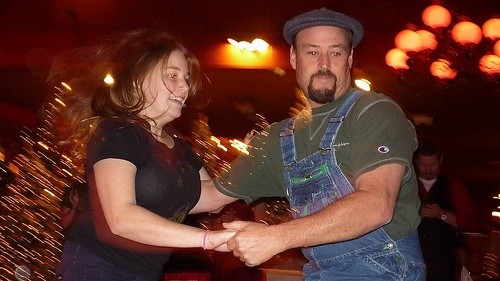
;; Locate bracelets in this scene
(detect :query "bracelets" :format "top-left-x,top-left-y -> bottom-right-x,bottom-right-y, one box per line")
202,228 -> 208,251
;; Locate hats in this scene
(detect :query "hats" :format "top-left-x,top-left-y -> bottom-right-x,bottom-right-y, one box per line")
283,7 -> 364,47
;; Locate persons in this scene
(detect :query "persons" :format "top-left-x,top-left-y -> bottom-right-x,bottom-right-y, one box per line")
54,30 -> 278,280
59,178 -> 87,247
412,144 -> 480,281
187,7 -> 428,281
